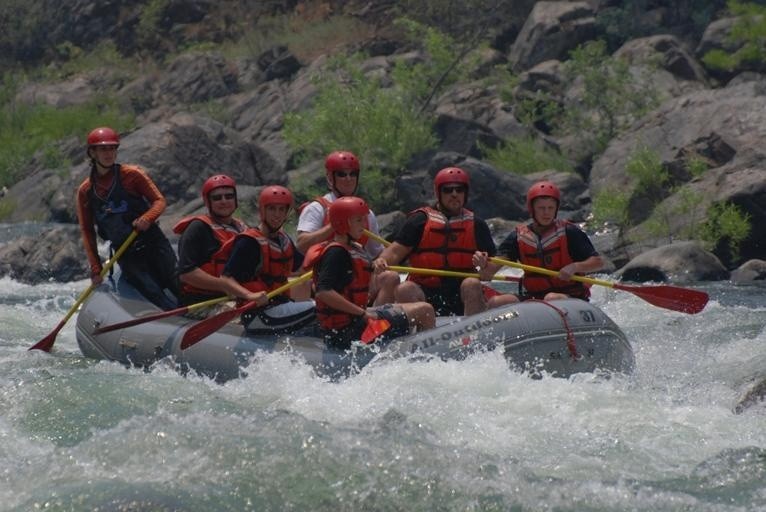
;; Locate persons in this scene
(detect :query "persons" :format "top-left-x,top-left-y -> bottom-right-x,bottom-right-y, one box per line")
218,185 -> 320,337
370,167 -> 493,321
76,126 -> 177,314
171,174 -> 250,320
296,150 -> 401,308
471,181 -> 605,311
312,194 -> 437,351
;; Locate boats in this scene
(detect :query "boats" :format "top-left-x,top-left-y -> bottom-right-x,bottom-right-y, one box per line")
77,281 -> 635,393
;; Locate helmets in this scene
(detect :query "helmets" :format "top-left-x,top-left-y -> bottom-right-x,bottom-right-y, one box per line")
85,127 -> 119,149
258,185 -> 294,224
201,174 -> 238,212
432,167 -> 472,204
324,150 -> 360,191
329,196 -> 370,237
526,179 -> 560,215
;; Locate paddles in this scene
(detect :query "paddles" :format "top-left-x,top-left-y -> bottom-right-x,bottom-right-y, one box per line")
484,256 -> 709,315
29,228 -> 137,351
93,295 -> 234,333
361,318 -> 390,346
181,269 -> 311,349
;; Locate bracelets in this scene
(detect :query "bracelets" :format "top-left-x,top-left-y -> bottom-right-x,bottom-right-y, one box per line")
360,311 -> 367,317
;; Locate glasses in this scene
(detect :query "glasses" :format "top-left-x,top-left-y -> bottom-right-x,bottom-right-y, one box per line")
334,170 -> 358,178
440,185 -> 467,195
210,192 -> 235,202
